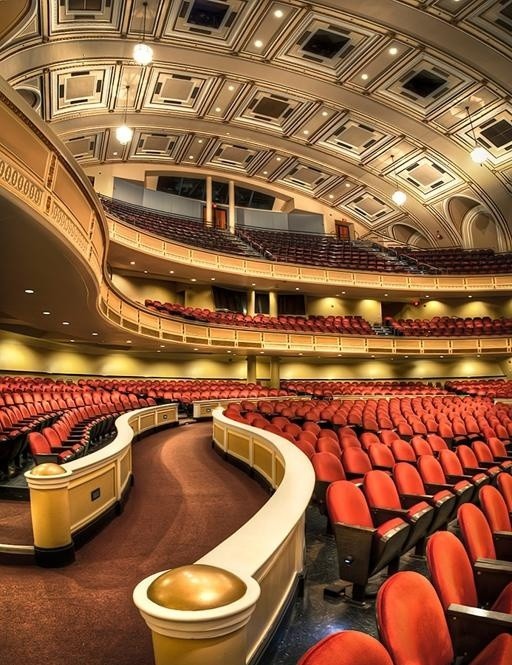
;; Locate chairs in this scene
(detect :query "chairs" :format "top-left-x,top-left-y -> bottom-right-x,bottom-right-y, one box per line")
95,194 -> 512,335
0,377 -> 512,665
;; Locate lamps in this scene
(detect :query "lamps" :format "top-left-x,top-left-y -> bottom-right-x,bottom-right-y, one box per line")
114,84 -> 136,146
390,154 -> 410,206
131,1 -> 155,68
465,106 -> 490,169
